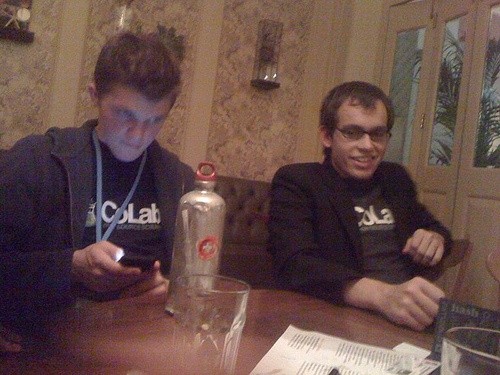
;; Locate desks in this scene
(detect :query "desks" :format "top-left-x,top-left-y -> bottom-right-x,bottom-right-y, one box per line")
0,279 -> 441,375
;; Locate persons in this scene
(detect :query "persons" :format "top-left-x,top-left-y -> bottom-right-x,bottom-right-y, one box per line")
0,30 -> 198,333
266,80 -> 446,331
0,323 -> 23,353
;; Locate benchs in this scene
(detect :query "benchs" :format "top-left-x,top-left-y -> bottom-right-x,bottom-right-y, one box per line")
188,173 -> 269,290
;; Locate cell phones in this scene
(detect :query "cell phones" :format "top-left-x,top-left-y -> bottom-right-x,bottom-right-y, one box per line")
116,254 -> 154,272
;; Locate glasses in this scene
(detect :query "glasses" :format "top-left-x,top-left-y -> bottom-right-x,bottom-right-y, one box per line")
333,124 -> 393,142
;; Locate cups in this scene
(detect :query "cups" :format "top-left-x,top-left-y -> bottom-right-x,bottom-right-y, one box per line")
440,327 -> 500,375
168,273 -> 251,375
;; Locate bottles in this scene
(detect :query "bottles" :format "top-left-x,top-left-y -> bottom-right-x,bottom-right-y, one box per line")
163,161 -> 226,317
